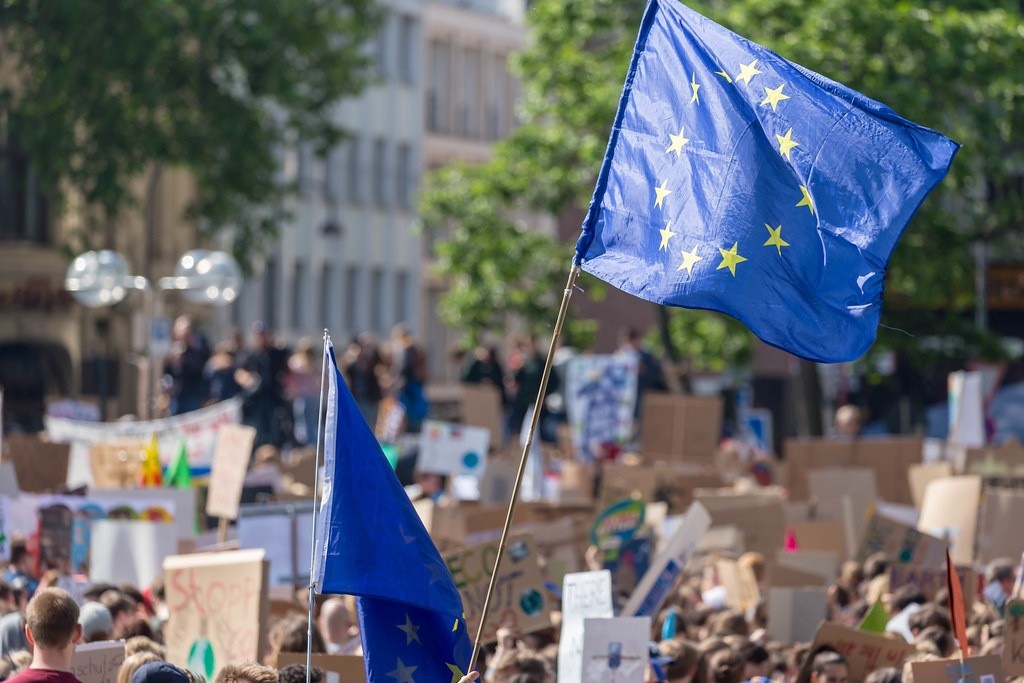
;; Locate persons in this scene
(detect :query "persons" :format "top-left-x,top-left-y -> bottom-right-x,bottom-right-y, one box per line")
1,304 -> 1024,502
0,535 -> 1024,682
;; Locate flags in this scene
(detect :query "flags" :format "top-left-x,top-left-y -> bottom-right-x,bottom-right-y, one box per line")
315,342 -> 484,683
161,435 -> 200,533
131,435 -> 173,524
577,0 -> 963,364
